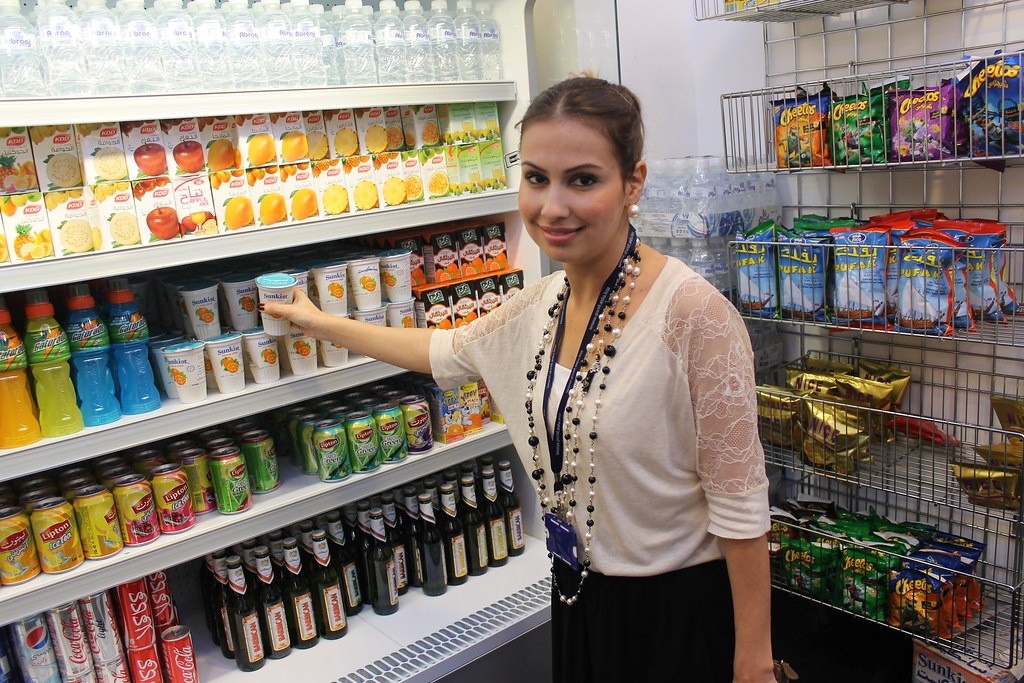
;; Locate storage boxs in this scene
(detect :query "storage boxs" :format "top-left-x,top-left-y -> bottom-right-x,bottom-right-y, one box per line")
912,590 -> 1024,683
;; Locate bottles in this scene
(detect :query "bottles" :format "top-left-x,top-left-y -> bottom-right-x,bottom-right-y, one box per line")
628,155 -> 783,396
1,279 -> 162,450
2,0 -> 503,99
202,454 -> 525,674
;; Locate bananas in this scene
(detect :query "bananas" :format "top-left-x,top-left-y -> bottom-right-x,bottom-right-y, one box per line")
16,162 -> 26,180
33,231 -> 42,245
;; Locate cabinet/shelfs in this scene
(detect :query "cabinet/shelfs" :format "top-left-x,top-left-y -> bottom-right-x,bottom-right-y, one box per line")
693,0 -> 1024,668
0,0 -> 551,683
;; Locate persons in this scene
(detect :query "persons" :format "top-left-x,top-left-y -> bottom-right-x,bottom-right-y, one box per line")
260,78 -> 775,683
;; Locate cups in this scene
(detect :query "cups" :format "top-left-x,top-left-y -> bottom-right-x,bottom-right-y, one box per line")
148,243 -> 423,407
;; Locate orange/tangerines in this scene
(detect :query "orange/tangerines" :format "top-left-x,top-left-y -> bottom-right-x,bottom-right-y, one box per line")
410,253 -> 509,328
446,413 -> 482,435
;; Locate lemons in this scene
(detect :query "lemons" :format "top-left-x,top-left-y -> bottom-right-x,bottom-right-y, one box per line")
92,226 -> 103,252
0,122 -> 117,145
0,182 -> 130,217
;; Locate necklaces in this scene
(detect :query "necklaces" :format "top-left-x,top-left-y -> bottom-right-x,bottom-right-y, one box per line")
525,235 -> 640,605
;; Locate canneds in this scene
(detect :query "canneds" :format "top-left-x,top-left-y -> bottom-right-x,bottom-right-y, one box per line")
1,384 -> 434,590
0,568 -> 201,683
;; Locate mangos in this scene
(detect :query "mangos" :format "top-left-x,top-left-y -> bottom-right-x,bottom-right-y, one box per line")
207,130 -> 318,231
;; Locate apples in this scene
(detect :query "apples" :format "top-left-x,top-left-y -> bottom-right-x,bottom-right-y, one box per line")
133,140 -> 215,240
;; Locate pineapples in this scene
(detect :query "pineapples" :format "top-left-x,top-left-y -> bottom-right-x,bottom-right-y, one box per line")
13,224 -> 36,258
0,154 -> 18,189
305,125 -> 405,214
